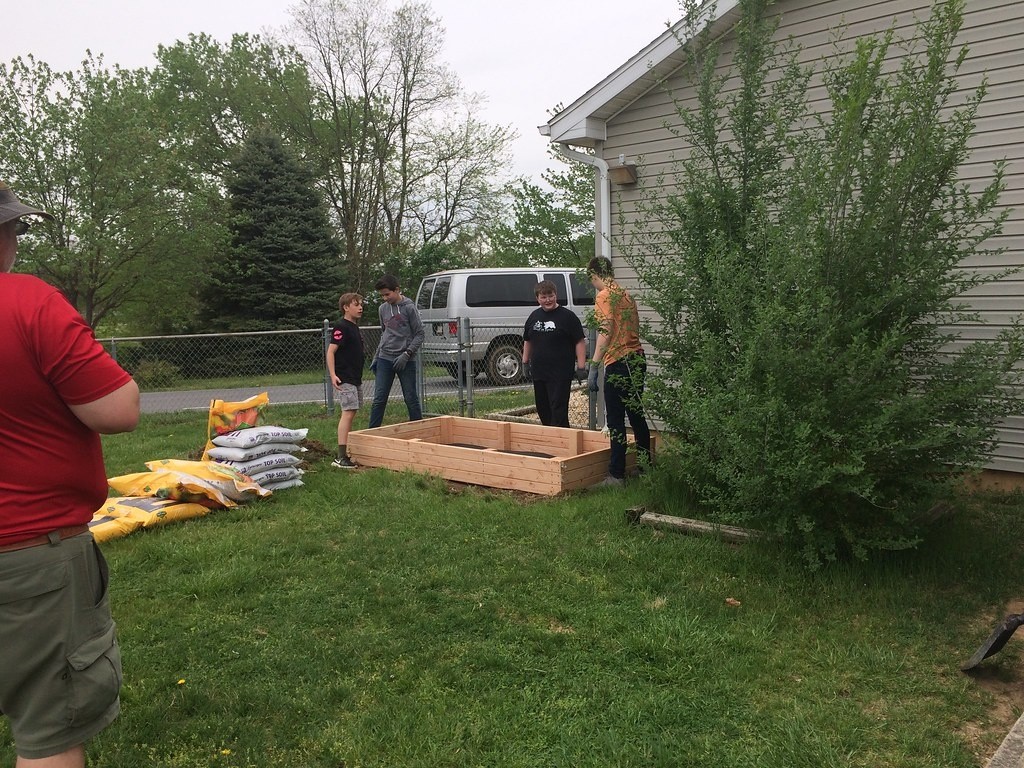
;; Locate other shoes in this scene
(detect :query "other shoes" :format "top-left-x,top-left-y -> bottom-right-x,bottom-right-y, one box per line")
628,469 -> 645,478
582,473 -> 624,494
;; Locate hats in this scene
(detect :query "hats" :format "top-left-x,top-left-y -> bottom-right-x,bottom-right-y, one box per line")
0,178 -> 55,225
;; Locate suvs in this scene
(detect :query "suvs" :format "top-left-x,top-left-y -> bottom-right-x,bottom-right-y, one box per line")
411,266 -> 604,389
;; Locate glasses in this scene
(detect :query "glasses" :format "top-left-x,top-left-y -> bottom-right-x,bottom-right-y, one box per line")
10,217 -> 30,236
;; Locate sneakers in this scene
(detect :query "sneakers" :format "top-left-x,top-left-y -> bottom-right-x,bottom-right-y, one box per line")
331,455 -> 358,469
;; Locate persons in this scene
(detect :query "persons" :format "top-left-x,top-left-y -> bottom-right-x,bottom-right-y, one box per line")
585,258 -> 652,489
369,275 -> 424,425
521,280 -> 587,428
326,293 -> 364,468
0,179 -> 141,768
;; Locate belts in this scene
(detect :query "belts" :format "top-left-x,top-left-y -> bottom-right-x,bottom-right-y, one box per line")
0,524 -> 89,553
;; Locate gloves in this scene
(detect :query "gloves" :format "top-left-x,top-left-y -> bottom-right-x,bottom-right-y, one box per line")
368,359 -> 377,376
393,352 -> 410,372
575,367 -> 588,385
588,362 -> 600,393
522,363 -> 533,383
